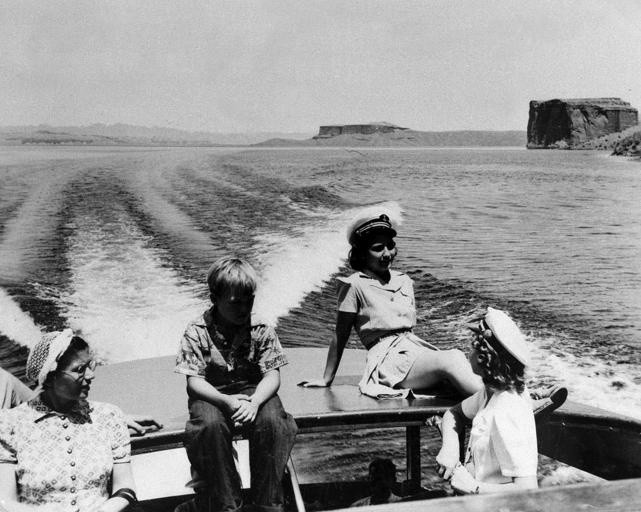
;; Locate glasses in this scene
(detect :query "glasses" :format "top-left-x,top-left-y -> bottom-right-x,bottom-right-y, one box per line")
58,360 -> 99,378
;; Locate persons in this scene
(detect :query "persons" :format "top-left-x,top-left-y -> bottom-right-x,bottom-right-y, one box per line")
1,367 -> 164,437
435,306 -> 540,495
172,255 -> 291,511
175,465 -> 210,511
349,458 -> 401,507
1,327 -> 136,511
296,213 -> 569,417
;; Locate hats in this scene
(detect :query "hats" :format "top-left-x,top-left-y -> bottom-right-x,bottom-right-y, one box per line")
467,306 -> 530,372
24,329 -> 77,390
347,207 -> 399,246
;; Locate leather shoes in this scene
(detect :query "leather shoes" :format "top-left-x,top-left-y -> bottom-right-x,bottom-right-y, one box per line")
531,386 -> 569,412
530,398 -> 555,417
